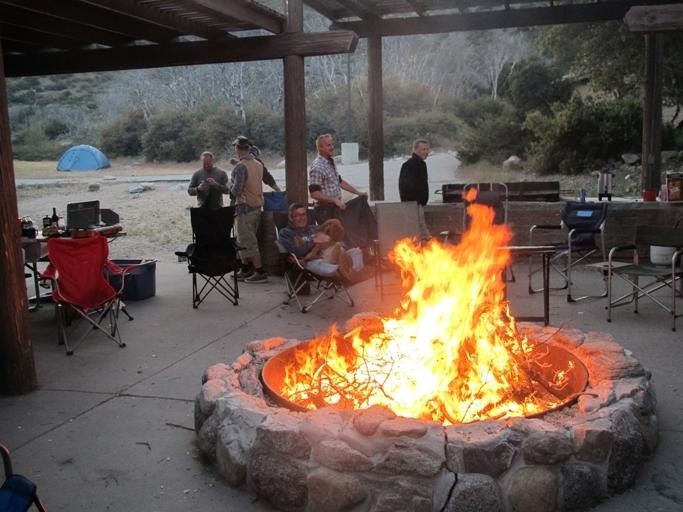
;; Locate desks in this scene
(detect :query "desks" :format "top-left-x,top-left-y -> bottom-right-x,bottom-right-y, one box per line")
21,220 -> 123,313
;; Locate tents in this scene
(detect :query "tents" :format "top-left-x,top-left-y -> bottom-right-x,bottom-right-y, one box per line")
57,144 -> 111,172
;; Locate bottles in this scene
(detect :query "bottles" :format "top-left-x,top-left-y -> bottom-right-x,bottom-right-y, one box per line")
58,216 -> 65,230
22,217 -> 27,224
580,188 -> 586,203
27,215 -> 32,224
43,215 -> 51,228
51,208 -> 57,226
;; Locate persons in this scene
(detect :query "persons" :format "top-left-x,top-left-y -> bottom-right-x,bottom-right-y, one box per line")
250,146 -> 282,192
188,151 -> 228,208
309,134 -> 368,250
399,139 -> 430,207
230,135 -> 269,284
278,202 -> 357,283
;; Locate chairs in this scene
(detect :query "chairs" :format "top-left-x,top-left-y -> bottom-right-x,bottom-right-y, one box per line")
462,182 -> 509,249
608,225 -> 683,332
527,202 -> 609,304
187,205 -> 240,307
40,236 -> 134,355
372,201 -> 422,301
273,210 -> 354,314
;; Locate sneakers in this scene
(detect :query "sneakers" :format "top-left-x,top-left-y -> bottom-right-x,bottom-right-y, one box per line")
244,272 -> 267,283
230,268 -> 252,280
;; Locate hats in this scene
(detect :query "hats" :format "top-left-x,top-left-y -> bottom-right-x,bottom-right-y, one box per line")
233,136 -> 248,148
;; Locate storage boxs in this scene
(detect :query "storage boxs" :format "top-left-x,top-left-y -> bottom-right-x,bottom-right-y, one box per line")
104,259 -> 156,301
